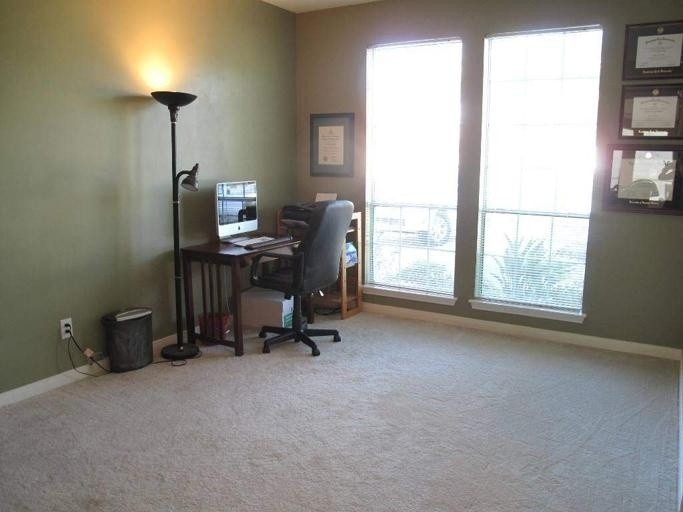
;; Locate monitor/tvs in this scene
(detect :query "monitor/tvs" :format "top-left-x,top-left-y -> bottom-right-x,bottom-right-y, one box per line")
215,179 -> 258,243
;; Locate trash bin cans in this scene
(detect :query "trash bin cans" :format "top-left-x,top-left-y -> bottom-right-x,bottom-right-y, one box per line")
100,307 -> 153,372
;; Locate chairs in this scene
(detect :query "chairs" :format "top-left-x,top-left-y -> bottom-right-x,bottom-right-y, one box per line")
247,199 -> 355,356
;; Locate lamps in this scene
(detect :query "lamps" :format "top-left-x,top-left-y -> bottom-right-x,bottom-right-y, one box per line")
151,90 -> 201,361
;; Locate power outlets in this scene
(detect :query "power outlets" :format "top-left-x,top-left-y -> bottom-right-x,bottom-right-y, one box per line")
59,317 -> 74,341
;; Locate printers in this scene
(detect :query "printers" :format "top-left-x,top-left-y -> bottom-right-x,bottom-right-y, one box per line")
280,193 -> 337,226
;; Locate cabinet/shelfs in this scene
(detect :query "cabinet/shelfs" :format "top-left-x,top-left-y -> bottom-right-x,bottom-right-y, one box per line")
274,206 -> 363,321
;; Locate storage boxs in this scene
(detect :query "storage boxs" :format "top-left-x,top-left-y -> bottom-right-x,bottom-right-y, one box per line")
237,286 -> 293,333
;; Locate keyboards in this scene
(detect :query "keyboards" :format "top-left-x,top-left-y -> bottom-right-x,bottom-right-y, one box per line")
233,236 -> 274,247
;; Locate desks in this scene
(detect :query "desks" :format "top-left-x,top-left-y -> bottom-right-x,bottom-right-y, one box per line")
178,232 -> 301,358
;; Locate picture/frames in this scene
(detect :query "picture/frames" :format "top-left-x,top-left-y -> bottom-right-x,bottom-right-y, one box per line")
601,142 -> 682,217
308,111 -> 355,178
616,82 -> 682,140
620,19 -> 682,81
